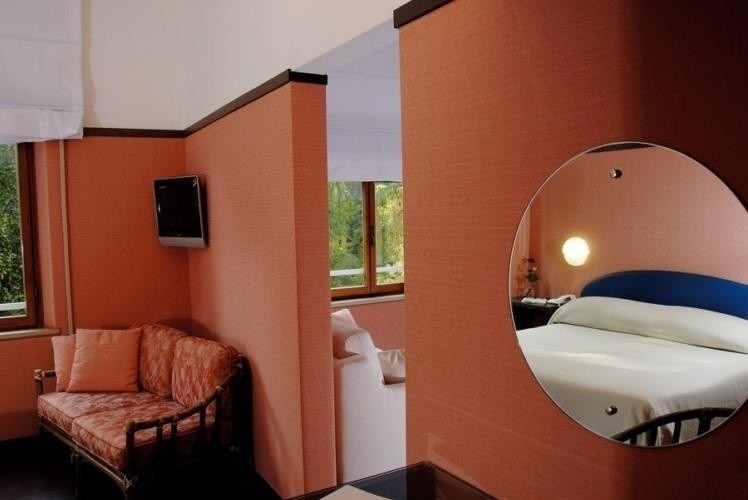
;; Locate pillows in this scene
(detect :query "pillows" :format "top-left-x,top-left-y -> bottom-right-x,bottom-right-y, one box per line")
50,322 -> 232,417
548,296 -> 748,361
331,308 -> 406,384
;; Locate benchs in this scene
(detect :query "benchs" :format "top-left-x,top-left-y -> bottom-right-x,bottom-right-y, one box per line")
34,356 -> 255,500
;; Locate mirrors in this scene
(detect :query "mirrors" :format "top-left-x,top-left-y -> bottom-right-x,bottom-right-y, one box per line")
507,142 -> 748,449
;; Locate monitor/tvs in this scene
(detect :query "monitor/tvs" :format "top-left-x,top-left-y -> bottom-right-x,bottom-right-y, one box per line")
152,174 -> 208,250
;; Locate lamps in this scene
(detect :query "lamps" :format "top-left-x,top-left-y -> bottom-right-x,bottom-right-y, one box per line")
560,233 -> 593,269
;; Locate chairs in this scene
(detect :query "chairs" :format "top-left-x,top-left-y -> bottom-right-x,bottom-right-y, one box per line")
330,353 -> 406,486
610,407 -> 735,447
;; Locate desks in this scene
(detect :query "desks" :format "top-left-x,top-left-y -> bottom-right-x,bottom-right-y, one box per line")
285,461 -> 502,500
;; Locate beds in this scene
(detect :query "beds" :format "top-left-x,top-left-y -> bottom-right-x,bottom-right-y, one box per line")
516,271 -> 748,447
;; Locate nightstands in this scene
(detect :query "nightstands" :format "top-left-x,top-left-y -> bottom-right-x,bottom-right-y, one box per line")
510,297 -> 564,330
